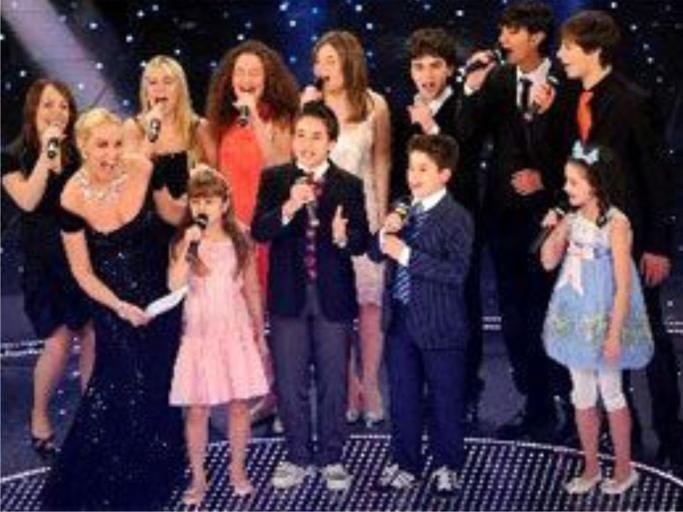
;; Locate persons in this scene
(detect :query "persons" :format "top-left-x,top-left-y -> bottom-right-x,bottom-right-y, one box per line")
538,137 -> 660,500
383,26 -> 483,436
250,99 -> 373,494
1,74 -> 95,461
460,2 -> 583,450
164,160 -> 269,506
205,36 -> 304,438
36,104 -> 253,511
300,28 -> 391,428
367,129 -> 478,495
121,46 -> 220,374
527,7 -> 683,479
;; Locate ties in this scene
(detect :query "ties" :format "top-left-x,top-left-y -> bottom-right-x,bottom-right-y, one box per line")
396,203 -> 422,304
519,78 -> 532,114
306,172 -> 318,282
577,91 -> 593,142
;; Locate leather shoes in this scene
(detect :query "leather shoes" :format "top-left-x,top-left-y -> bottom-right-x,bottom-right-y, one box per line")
497,394 -> 574,442
653,422 -> 683,466
345,388 -> 384,424
565,465 -> 644,496
30,413 -> 56,453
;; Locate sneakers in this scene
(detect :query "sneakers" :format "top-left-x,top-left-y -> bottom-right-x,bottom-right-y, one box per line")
272,461 -> 314,489
227,463 -> 253,495
377,462 -> 417,492
249,392 -> 284,433
322,461 -> 351,492
181,473 -> 210,505
430,467 -> 461,495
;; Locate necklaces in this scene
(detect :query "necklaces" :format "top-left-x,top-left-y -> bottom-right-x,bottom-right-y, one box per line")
76,166 -> 129,206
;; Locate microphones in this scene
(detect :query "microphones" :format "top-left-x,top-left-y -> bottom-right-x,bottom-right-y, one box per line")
291,168 -> 321,229
412,93 -> 423,138
45,137 -> 58,159
147,118 -> 161,142
519,69 -> 563,126
386,197 -> 412,235
187,214 -> 209,258
311,76 -> 324,93
527,200 -> 568,255
455,47 -> 507,74
236,104 -> 249,128
